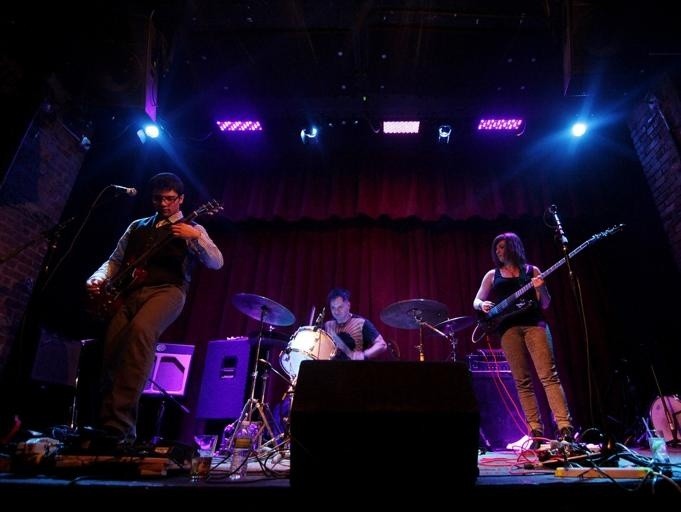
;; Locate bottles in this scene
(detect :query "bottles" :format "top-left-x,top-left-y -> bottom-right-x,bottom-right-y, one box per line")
229,421 -> 252,480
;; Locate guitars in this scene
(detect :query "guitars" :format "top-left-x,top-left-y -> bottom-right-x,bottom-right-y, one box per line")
477,224 -> 623,335
90,199 -> 224,317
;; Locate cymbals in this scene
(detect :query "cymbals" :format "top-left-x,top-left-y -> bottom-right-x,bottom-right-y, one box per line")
229,293 -> 294,326
427,315 -> 474,336
379,299 -> 451,329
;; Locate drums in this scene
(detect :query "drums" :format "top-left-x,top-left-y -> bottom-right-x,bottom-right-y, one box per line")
649,394 -> 681,443
280,325 -> 337,378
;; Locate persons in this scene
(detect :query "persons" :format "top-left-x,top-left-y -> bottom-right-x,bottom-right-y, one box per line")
321,288 -> 388,361
472,232 -> 574,450
84,172 -> 225,447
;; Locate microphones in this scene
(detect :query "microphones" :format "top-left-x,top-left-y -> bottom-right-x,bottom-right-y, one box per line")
549,205 -> 569,244
113,185 -> 137,196
180,404 -> 191,415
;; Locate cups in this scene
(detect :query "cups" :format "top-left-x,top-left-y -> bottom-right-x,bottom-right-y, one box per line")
195,434 -> 219,477
647,429 -> 672,471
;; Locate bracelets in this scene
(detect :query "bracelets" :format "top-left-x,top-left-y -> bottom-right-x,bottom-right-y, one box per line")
479,304 -> 482,311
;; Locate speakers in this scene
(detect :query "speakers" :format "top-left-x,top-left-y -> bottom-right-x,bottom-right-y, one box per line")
581,425 -> 623,466
289,361 -> 480,486
195,336 -> 267,420
471,371 -> 538,449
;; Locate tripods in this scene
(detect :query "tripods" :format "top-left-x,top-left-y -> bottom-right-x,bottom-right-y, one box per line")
218,309 -> 285,455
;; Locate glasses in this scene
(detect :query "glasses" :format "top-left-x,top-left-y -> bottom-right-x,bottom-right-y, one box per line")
151,195 -> 181,205
